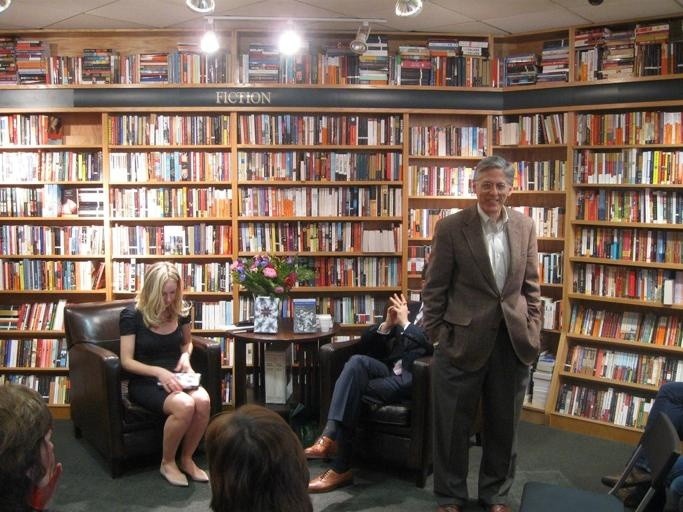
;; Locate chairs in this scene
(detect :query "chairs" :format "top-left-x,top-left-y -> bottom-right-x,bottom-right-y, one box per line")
514,411 -> 681,512
57,298 -> 224,481
313,302 -> 484,488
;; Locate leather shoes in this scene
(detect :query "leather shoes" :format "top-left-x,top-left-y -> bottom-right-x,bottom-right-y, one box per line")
488,504 -> 510,512
304,435 -> 340,459
308,468 -> 354,493
179,464 -> 209,482
160,464 -> 189,487
437,505 -> 458,512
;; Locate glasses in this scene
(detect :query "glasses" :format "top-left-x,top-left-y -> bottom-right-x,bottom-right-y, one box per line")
481,182 -> 508,194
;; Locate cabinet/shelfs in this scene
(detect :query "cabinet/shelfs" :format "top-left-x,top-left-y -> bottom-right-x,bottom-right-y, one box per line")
405,101 -> 681,447
0,25 -> 234,90
106,105 -> 236,414
0,106 -> 108,423
233,28 -> 493,90
493,11 -> 683,94
234,107 -> 408,416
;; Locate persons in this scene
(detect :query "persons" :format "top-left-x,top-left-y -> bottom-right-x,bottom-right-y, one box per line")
120,261 -> 210,486
0,385 -> 62,511
305,263 -> 433,491
207,405 -> 313,512
425,157 -> 541,512
602,382 -> 681,512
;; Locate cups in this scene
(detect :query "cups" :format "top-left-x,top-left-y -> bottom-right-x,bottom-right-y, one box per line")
318,315 -> 330,332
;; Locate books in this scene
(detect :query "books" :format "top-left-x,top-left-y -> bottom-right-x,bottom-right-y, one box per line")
236,111 -> 403,333
493,112 -> 569,191
511,205 -> 563,408
408,125 -> 488,241
407,247 -> 429,312
187,299 -> 234,404
108,113 -> 231,293
0,25 -> 683,90
0,113 -> 105,405
555,113 -> 682,430
156,371 -> 201,388
